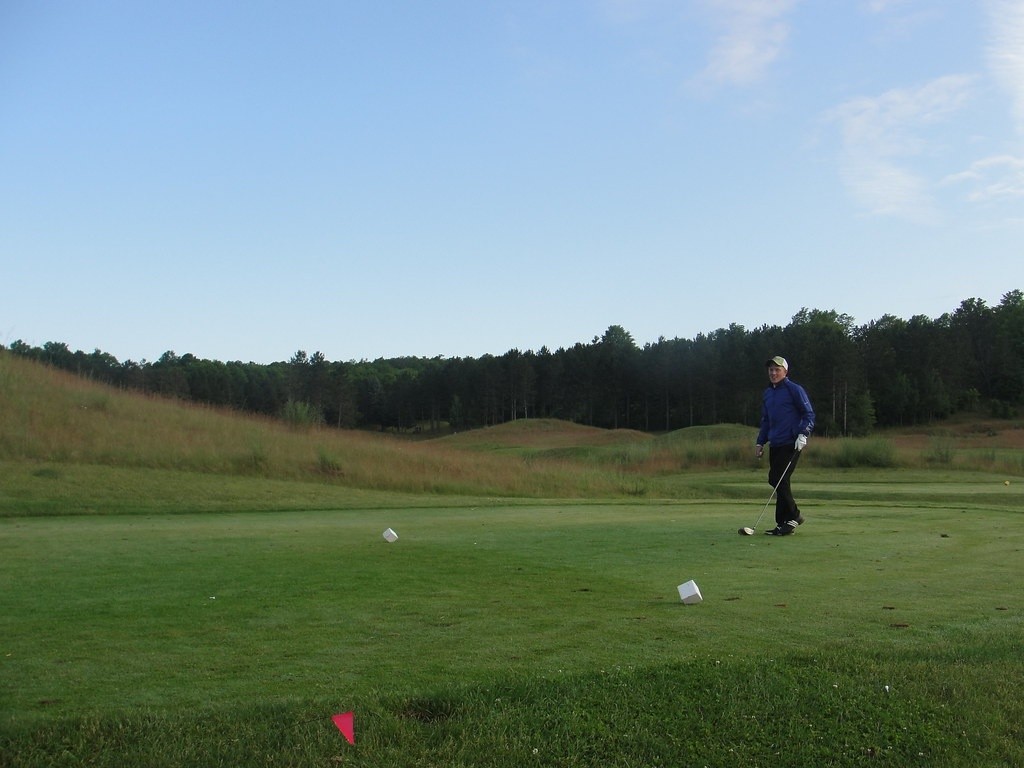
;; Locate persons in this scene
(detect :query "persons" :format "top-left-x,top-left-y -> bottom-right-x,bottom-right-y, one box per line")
754,356 -> 815,536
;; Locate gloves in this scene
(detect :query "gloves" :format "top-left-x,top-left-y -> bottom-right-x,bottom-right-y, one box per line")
794,433 -> 807,451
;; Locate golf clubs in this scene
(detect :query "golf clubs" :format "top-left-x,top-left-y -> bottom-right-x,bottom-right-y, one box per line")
737,449 -> 799,536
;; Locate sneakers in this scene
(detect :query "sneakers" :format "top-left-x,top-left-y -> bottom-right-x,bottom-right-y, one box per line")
780,513 -> 805,532
765,525 -> 795,535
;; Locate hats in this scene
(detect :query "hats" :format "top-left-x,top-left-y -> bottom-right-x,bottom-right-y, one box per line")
767,356 -> 788,371
